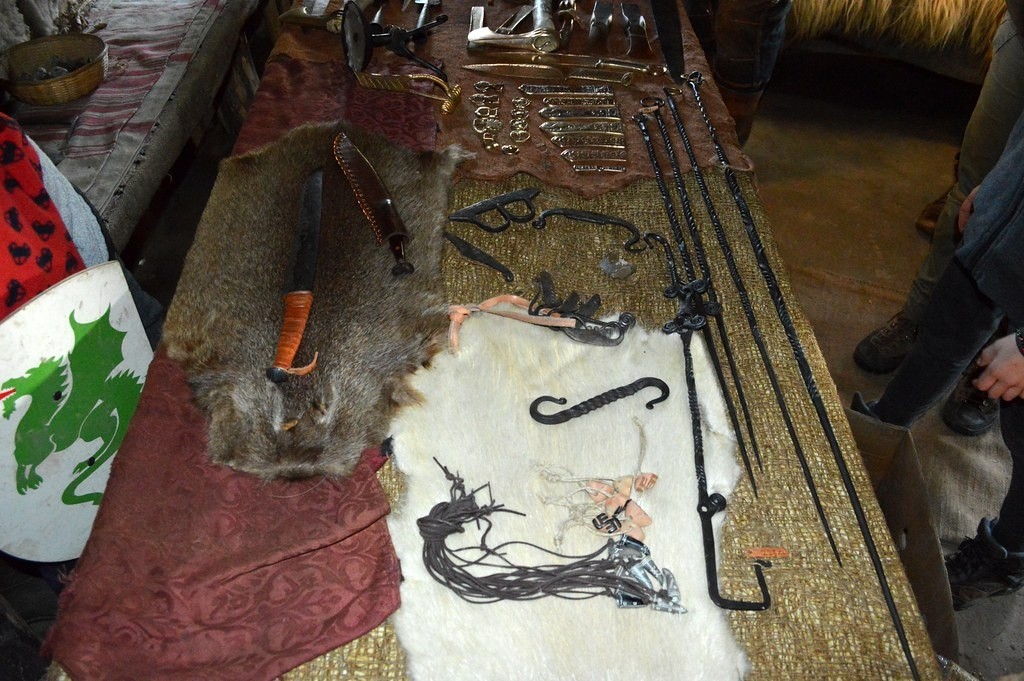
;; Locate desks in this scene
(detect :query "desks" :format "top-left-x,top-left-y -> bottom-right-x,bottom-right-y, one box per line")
43,0 -> 943,680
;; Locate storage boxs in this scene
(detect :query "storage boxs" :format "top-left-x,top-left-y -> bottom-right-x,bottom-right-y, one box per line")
842,407 -> 960,666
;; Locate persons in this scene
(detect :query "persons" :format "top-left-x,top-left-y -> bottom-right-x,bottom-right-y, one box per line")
0,112 -> 166,643
850,110 -> 1024,610
853,0 -> 1024,435
713,0 -> 793,148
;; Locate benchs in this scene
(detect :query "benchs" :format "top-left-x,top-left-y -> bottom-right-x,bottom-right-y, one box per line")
0,1 -> 281,255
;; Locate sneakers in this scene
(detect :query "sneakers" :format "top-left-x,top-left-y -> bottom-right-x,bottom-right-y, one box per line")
854,311 -> 918,375
942,359 -> 1000,436
943,517 -> 1024,612
850,391 -> 880,419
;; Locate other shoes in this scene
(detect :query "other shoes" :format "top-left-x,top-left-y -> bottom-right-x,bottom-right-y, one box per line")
916,181 -> 956,235
